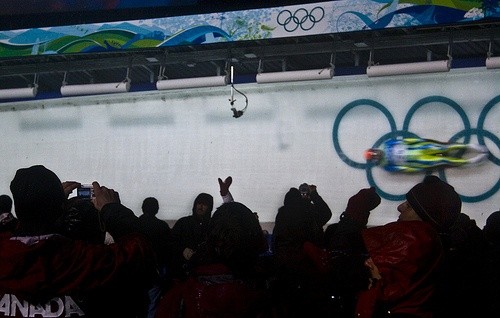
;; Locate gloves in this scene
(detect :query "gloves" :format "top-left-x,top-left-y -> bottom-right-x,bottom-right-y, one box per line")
345,188 -> 381,218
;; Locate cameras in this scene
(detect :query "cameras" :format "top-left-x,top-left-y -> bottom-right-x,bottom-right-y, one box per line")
77,186 -> 95,199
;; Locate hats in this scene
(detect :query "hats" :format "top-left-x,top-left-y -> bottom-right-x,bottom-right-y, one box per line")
406,175 -> 462,224
10,165 -> 64,219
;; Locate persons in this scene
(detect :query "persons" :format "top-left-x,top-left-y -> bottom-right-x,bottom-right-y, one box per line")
365,137 -> 489,175
0,164 -> 500,318
